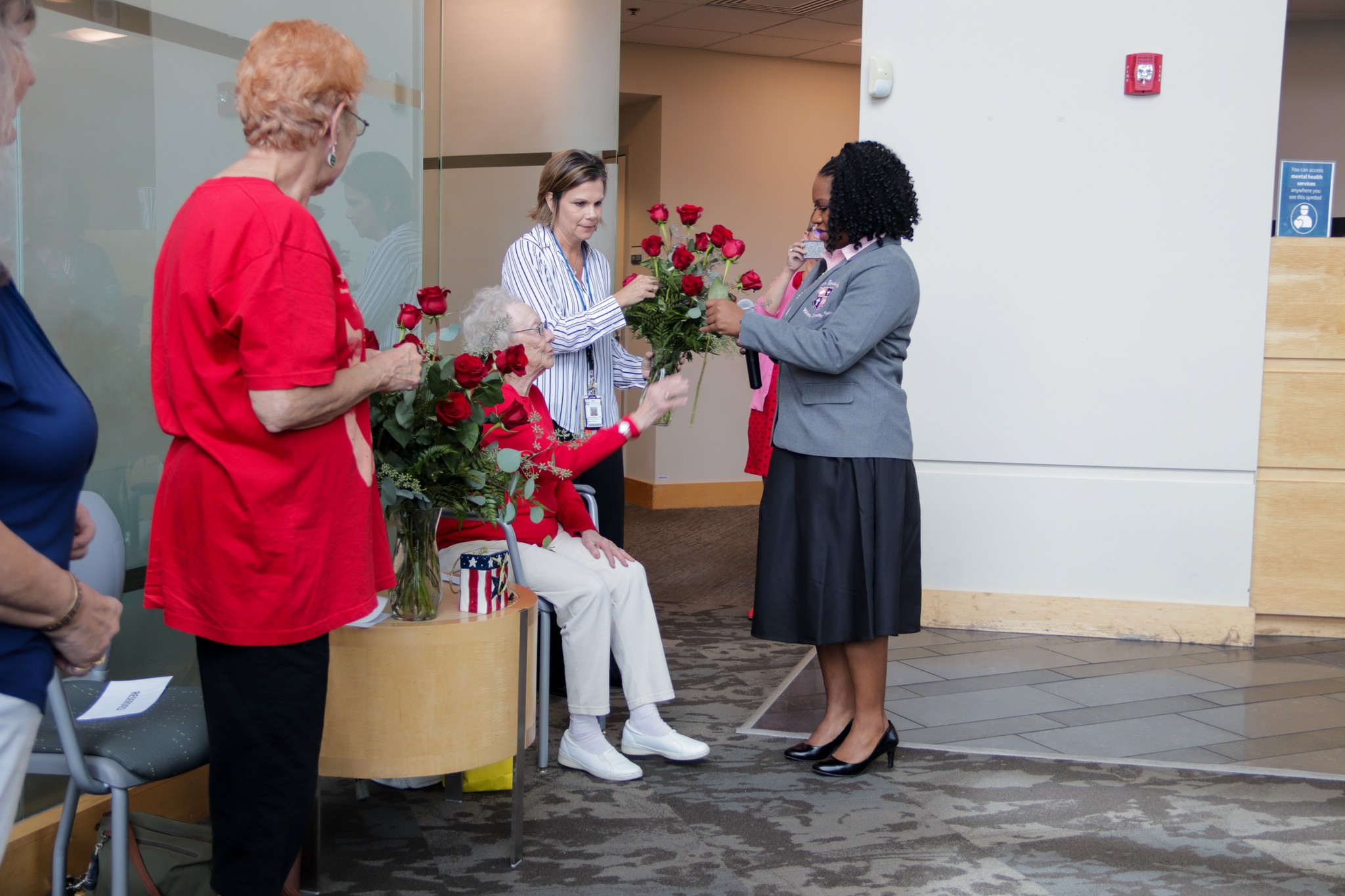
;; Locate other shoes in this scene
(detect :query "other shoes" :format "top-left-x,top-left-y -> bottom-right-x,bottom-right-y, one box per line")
555,728 -> 644,781
620,719 -> 710,762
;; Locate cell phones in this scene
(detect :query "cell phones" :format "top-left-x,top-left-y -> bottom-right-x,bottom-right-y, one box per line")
802,240 -> 827,259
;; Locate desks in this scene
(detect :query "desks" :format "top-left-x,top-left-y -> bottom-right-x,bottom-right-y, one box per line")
297,580 -> 539,896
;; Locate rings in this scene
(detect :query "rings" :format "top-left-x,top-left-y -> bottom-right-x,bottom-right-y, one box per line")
75,665 -> 93,670
790,248 -> 794,252
91,653 -> 106,666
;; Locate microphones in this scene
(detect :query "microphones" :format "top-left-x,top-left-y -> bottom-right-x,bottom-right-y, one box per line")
737,299 -> 762,389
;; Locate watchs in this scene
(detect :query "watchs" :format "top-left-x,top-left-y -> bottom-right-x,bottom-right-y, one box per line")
617,419 -> 632,441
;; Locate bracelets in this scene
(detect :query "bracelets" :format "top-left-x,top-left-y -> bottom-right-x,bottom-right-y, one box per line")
39,569 -> 83,632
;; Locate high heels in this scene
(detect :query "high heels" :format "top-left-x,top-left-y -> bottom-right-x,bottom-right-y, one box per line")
810,719 -> 899,777
783,715 -> 858,761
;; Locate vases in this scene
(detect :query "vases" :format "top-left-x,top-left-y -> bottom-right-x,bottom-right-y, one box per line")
639,346 -> 686,427
391,506 -> 444,623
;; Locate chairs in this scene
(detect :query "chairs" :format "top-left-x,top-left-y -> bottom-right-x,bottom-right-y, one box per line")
439,483 -> 608,775
23,491 -> 214,895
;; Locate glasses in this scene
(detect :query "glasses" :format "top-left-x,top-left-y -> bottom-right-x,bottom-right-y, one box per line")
808,227 -> 820,240
338,100 -> 369,136
512,319 -> 548,336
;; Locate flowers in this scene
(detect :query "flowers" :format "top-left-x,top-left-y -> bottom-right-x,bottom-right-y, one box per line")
365,285 -> 592,622
622,204 -> 763,425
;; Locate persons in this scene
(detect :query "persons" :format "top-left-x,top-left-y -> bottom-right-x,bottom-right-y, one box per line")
342,151 -> 421,351
500,148 -> 688,551
735,206 -> 836,620
0,0 -> 124,866
700,140 -> 922,778
437,285 -> 711,782
141,19 -> 421,896
